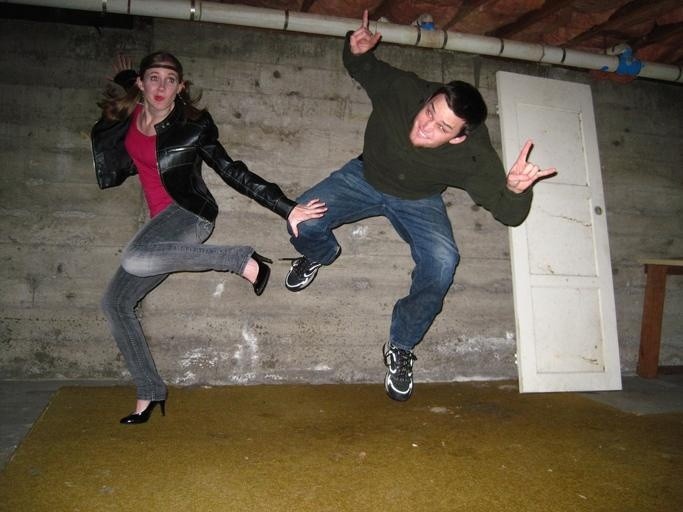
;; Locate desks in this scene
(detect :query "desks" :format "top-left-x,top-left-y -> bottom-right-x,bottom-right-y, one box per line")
636,258 -> 683,380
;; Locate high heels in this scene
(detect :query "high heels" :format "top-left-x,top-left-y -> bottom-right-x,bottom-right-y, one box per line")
120,390 -> 167,424
252,246 -> 273,296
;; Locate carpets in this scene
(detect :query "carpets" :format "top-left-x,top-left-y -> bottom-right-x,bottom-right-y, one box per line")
1,386 -> 682,511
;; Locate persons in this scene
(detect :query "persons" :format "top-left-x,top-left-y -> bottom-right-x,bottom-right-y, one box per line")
87,49 -> 329,429
279,7 -> 561,403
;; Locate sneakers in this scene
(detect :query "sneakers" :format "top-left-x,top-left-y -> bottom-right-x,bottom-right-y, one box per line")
381,338 -> 418,402
284,242 -> 341,293
